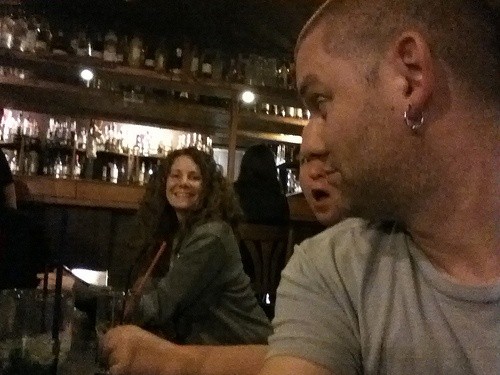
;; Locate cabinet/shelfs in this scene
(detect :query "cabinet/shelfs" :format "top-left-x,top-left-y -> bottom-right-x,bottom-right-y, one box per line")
0,48 -> 317,290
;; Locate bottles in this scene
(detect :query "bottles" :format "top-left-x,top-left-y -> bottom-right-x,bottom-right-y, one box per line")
0,10 -> 305,194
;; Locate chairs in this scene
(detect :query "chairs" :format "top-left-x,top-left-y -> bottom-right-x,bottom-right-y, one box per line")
0,205 -> 70,375
236,225 -> 294,310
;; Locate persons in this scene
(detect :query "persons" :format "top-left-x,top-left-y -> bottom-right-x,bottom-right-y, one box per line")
0,107 -> 18,209
36,146 -> 274,346
233,144 -> 291,322
101,116 -> 354,375
258,0 -> 500,375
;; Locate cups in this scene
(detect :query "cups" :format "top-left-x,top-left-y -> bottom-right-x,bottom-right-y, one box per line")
2,287 -> 74,366
95,286 -> 144,367
